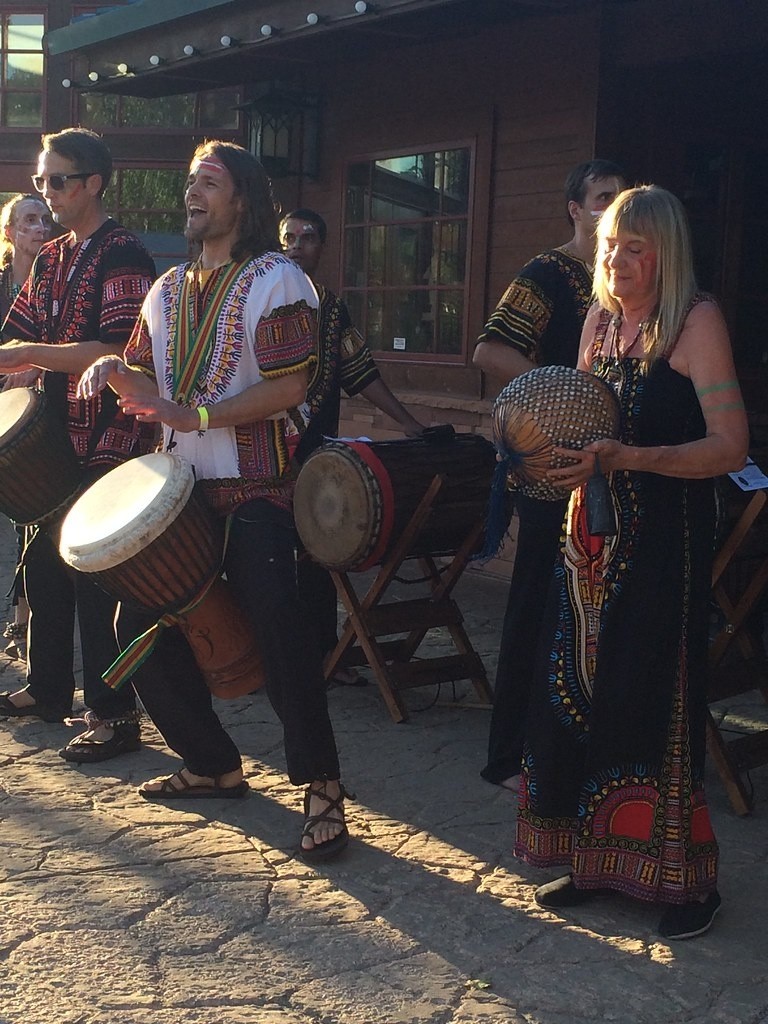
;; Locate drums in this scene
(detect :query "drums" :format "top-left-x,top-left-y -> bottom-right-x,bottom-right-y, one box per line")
58,454 -> 275,706
0,386 -> 82,524
294,435 -> 514,572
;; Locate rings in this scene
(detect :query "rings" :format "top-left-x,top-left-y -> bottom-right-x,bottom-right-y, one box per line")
575,483 -> 580,487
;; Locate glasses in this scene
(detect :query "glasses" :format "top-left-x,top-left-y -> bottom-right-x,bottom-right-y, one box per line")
32,169 -> 107,191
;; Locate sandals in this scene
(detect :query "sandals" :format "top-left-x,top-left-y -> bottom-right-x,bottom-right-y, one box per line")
138,763 -> 249,801
61,712 -> 144,764
0,682 -> 71,723
302,785 -> 358,864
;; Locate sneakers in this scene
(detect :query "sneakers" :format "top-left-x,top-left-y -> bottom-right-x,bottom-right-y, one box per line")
535,870 -> 608,904
656,886 -> 720,939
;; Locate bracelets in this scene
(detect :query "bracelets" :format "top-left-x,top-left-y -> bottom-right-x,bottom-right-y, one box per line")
197,407 -> 209,431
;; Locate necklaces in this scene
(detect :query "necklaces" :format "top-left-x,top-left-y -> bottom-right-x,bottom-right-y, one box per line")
612,310 -> 649,366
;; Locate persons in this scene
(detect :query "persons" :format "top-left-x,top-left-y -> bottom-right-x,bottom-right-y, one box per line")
473,161 -> 748,938
276,209 -> 424,686
75,141 -> 352,862
0,125 -> 158,763
